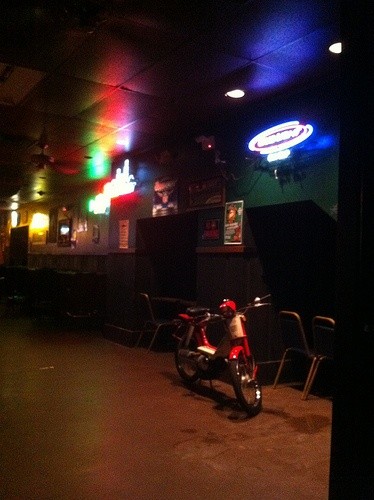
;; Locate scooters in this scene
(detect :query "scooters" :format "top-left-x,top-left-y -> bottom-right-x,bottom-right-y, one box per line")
170,293 -> 272,417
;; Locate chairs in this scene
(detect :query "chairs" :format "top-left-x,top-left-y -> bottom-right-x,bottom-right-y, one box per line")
272,312 -> 336,398
4,265 -> 101,333
135,292 -> 173,355
